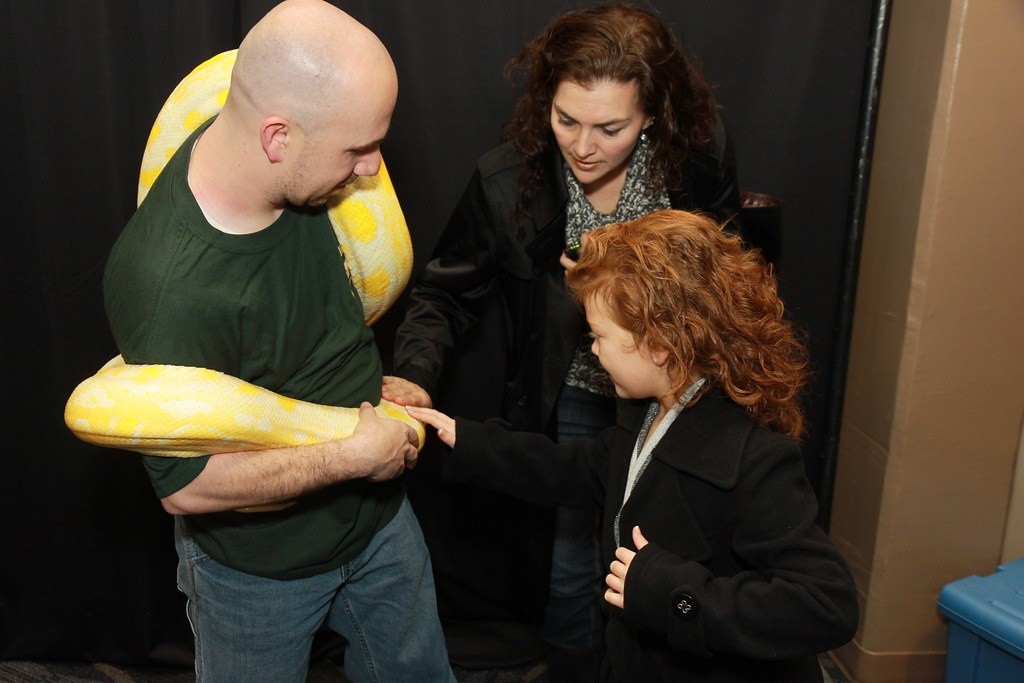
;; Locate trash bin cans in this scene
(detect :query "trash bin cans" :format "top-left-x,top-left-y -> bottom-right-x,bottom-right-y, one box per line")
937,556 -> 1023,683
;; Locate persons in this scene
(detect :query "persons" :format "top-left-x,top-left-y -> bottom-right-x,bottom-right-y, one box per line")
407,209 -> 858,683
395,0 -> 743,615
107,0 -> 454,683
